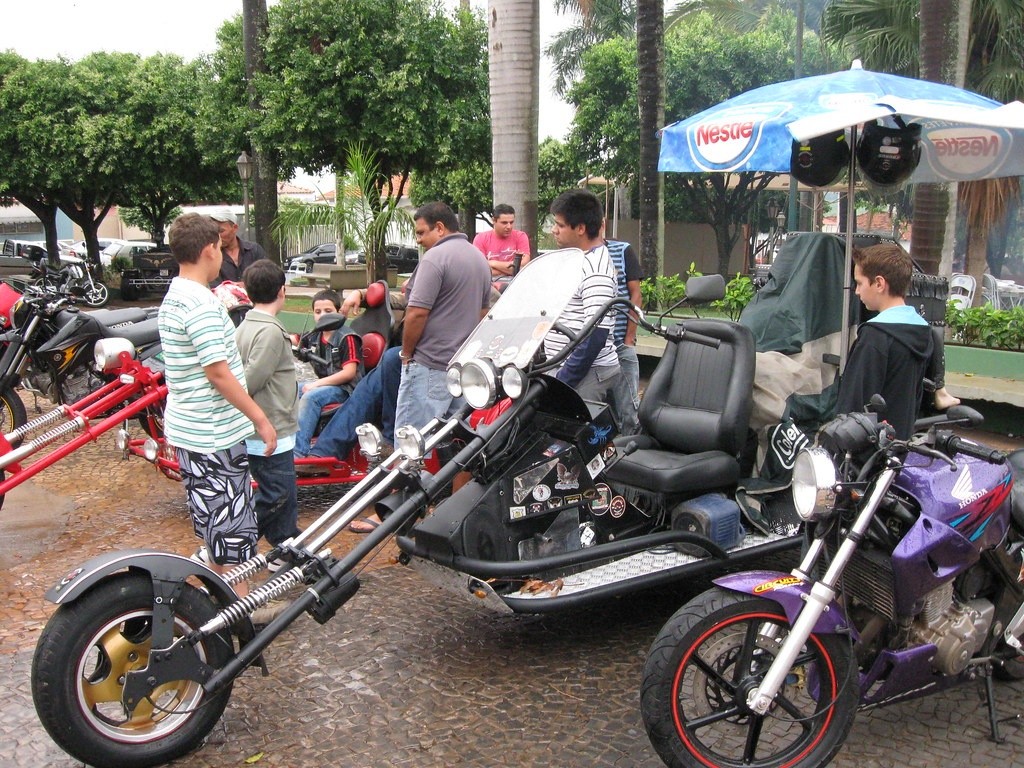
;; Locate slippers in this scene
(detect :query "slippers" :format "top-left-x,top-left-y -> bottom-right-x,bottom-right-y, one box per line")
348,517 -> 380,532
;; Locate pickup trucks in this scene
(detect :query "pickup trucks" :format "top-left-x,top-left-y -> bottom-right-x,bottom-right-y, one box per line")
0,239 -> 84,280
344,245 -> 419,274
283,243 -> 359,273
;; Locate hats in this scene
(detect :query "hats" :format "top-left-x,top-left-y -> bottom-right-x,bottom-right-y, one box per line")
209,207 -> 237,225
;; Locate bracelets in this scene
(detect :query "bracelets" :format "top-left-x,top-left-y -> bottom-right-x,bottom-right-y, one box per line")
398,350 -> 410,360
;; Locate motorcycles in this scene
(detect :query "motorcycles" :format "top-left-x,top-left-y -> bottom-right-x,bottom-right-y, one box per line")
25,242 -> 110,309
30,228 -> 946,768
638,390 -> 1024,768
0,274 -> 413,507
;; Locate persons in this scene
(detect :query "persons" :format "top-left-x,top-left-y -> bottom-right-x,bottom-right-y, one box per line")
159,213 -> 293,632
290,290 -> 364,460
600,216 -> 642,436
836,244 -> 944,462
192,259 -> 303,570
473,204 -> 531,291
543,190 -> 621,402
295,281 -> 500,476
211,210 -> 266,324
350,202 -> 492,533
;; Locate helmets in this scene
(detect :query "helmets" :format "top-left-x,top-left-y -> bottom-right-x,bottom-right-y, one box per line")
791,128 -> 850,190
857,119 -> 922,195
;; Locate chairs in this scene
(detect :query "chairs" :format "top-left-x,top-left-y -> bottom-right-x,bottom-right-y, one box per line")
597,319 -> 761,529
316,280 -> 400,436
944,272 -> 1024,317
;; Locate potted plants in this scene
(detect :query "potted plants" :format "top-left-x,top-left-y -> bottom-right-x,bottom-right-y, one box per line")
940,300 -> 1024,408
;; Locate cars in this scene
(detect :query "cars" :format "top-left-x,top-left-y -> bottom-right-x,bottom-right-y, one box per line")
71,238 -> 127,260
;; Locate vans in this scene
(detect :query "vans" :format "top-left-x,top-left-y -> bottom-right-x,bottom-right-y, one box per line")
99,241 -> 157,266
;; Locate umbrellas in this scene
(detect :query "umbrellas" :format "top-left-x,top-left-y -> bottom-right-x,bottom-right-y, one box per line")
658,60 -> 1024,378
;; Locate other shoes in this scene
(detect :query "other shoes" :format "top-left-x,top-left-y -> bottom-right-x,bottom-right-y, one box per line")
294,455 -> 343,474
249,599 -> 291,630
190,546 -> 212,567
268,547 -> 332,572
